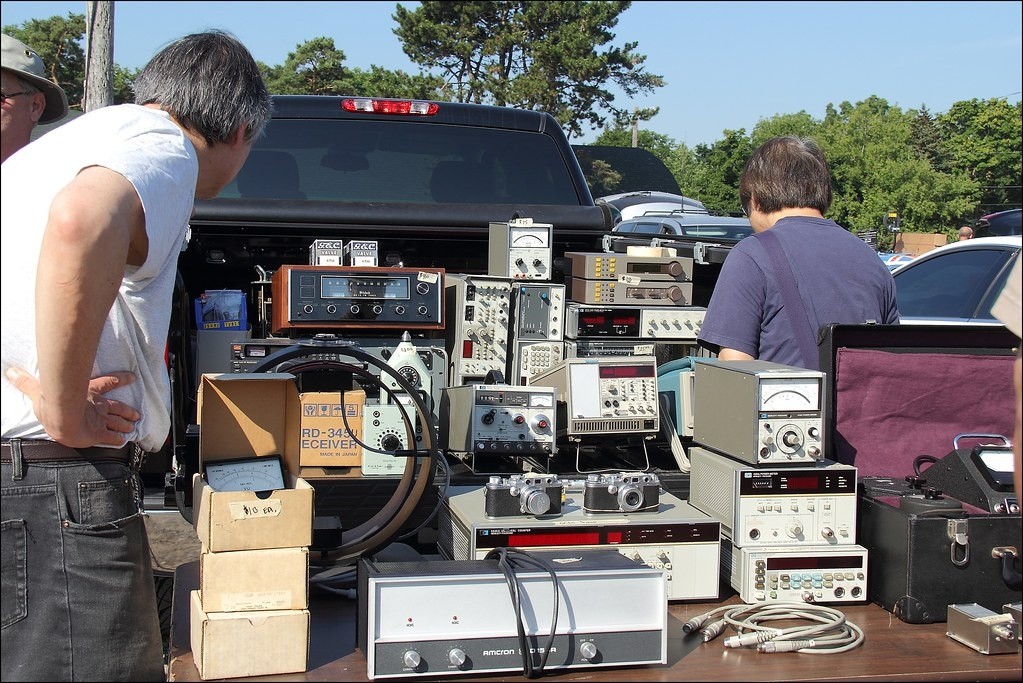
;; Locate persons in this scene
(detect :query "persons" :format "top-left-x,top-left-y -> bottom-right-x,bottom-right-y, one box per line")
957,227 -> 973,242
990,249 -> 1023,514
1,33 -> 69,166
1,31 -> 272,682
699,136 -> 901,373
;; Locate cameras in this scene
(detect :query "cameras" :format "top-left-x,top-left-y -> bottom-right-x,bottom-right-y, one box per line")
583,471 -> 662,514
484,472 -> 563,518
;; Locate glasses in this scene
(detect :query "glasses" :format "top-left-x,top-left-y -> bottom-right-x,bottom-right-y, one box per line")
0,91 -> 33,105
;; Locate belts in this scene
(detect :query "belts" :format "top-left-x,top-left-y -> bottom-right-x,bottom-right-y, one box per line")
1,442 -> 144,471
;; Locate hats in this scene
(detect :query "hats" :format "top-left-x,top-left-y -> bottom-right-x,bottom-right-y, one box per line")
1,33 -> 68,125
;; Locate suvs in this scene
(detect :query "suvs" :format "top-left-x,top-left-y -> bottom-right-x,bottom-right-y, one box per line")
139,95 -> 608,476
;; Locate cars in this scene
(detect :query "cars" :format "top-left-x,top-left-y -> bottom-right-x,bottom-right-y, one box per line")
595,191 -> 755,239
874,209 -> 1022,327
